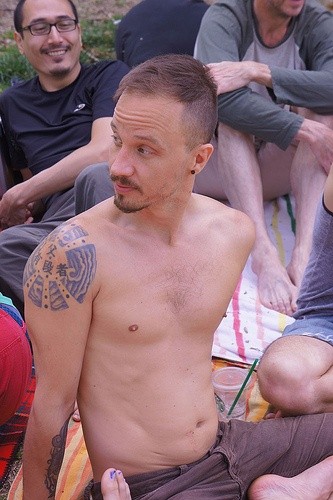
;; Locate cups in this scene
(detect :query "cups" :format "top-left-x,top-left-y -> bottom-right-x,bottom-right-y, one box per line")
211,367 -> 251,422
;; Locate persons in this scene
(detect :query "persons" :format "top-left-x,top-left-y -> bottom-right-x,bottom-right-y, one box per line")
21,54 -> 333,500
114,0 -> 215,66
0,0 -> 130,319
0,289 -> 33,424
258,163 -> 333,416
192,1 -> 333,316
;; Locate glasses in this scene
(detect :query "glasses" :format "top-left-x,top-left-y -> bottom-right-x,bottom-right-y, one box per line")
21,18 -> 77,35
